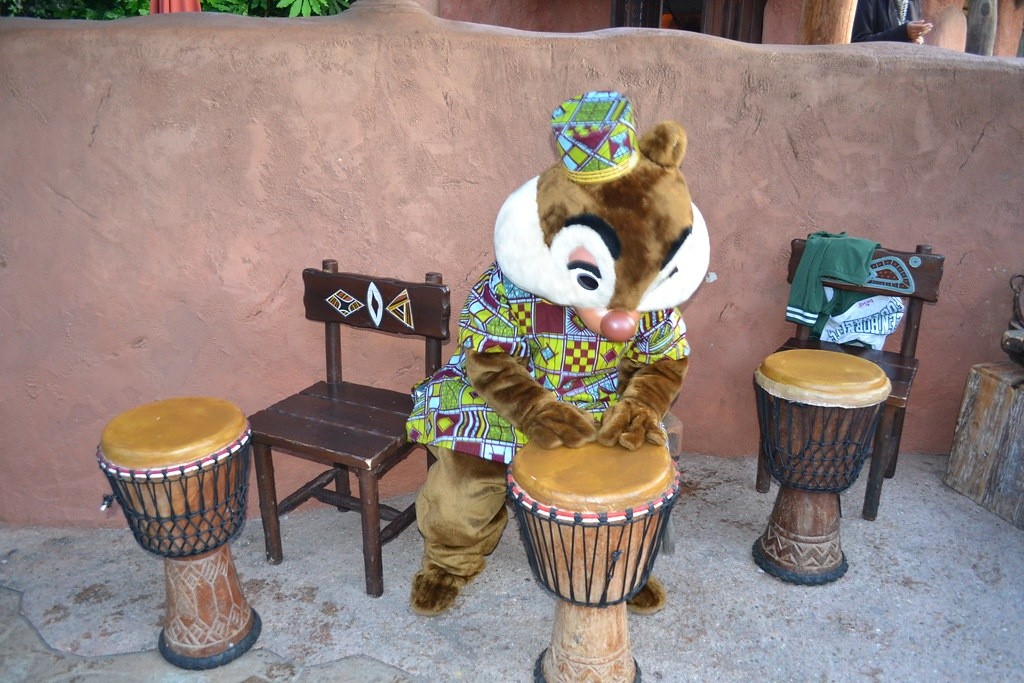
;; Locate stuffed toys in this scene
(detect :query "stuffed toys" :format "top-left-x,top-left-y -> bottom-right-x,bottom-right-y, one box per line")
406,90 -> 712,617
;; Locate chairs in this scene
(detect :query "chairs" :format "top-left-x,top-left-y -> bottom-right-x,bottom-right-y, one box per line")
754,235 -> 946,520
241,258 -> 453,598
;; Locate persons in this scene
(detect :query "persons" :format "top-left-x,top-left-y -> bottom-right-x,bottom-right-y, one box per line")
656,0 -> 681,30
852,0 -> 933,45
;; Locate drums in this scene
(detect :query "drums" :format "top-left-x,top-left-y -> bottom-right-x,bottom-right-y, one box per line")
505,430 -> 679,683
92,398 -> 264,670
750,346 -> 891,590
